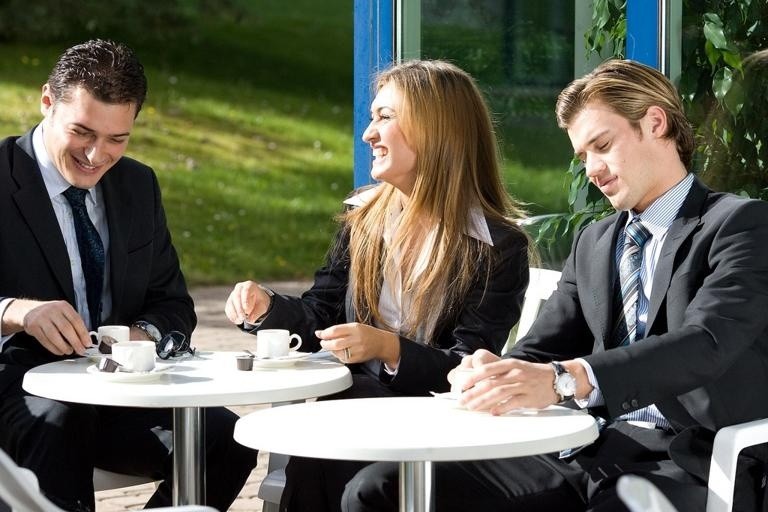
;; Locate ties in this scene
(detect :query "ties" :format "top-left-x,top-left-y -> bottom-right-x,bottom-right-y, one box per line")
609,219 -> 652,348
61,183 -> 105,345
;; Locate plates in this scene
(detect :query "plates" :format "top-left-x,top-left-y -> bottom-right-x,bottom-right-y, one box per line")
81,348 -> 177,382
248,352 -> 310,367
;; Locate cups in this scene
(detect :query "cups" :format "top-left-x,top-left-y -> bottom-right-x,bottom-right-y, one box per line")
111,341 -> 156,373
256,329 -> 302,358
236,355 -> 253,370
87,325 -> 130,355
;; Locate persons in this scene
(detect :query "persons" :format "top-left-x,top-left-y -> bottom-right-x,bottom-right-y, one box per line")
341,59 -> 768,512
225,58 -> 545,512
0,38 -> 259,512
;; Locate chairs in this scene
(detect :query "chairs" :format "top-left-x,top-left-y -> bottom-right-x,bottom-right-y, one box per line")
258,266 -> 562,512
704,419 -> 768,512
0,449 -> 219,512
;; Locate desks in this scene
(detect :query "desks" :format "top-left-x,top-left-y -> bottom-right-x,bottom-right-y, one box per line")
22,351 -> 353,512
233,397 -> 600,512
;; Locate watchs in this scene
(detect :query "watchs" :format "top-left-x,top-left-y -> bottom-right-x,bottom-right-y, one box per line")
549,359 -> 577,404
130,322 -> 162,342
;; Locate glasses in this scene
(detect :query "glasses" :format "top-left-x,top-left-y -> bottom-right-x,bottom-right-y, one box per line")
158,330 -> 195,360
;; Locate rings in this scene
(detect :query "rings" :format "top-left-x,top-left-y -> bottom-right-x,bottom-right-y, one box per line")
344,347 -> 353,360
